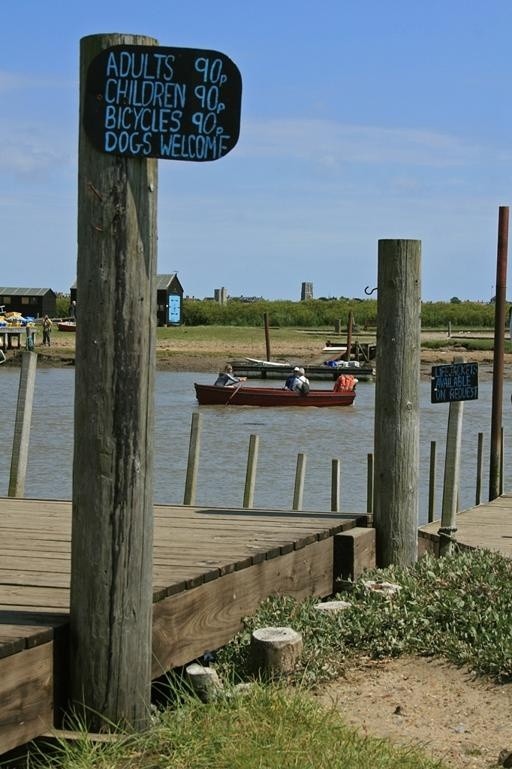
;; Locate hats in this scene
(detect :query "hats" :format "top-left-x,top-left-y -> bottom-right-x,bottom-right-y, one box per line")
294,367 -> 305,373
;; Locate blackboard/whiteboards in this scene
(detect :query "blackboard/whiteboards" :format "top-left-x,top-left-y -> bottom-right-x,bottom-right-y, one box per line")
431,361 -> 479,404
83,44 -> 242,162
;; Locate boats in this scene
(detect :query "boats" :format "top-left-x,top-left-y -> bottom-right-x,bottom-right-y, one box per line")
57,321 -> 77,333
227,344 -> 376,382
319,339 -> 376,354
192,373 -> 358,407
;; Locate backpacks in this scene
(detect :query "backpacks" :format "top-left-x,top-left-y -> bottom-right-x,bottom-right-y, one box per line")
296,379 -> 309,393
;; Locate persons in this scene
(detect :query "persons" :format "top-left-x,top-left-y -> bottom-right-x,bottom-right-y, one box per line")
42,315 -> 53,347
214,364 -> 246,389
284,367 -> 310,392
